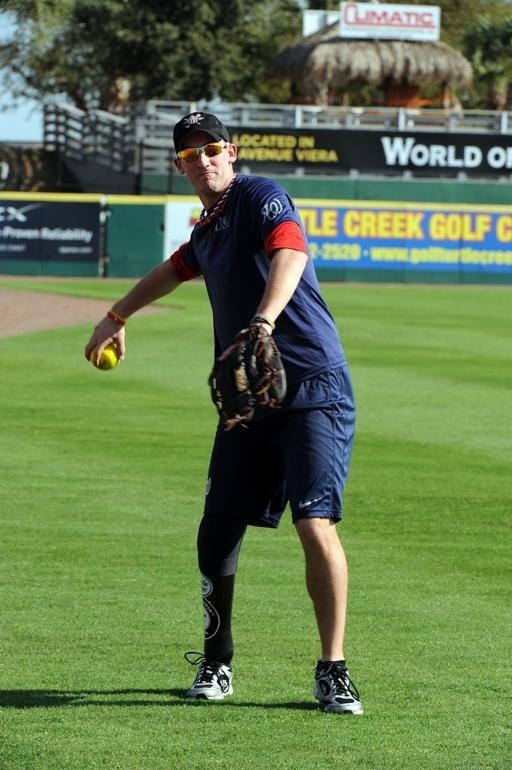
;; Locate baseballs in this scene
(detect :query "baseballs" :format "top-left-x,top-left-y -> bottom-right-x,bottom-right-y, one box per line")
91,345 -> 119,370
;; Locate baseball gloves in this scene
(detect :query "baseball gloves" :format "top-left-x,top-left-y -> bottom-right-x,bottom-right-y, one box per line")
208,325 -> 288,430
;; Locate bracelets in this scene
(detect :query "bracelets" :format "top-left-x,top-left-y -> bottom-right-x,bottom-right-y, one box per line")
107,309 -> 126,327
250,313 -> 276,330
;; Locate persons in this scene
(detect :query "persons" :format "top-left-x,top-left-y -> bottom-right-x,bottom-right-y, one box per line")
84,113 -> 362,717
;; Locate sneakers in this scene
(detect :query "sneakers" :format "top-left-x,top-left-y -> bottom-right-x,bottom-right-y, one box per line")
185,652 -> 233,700
313,659 -> 363,715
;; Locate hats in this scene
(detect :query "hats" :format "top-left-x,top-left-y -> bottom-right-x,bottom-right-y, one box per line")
173,112 -> 230,153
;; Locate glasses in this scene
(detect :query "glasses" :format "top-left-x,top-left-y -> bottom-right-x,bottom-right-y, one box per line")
176,142 -> 230,163
